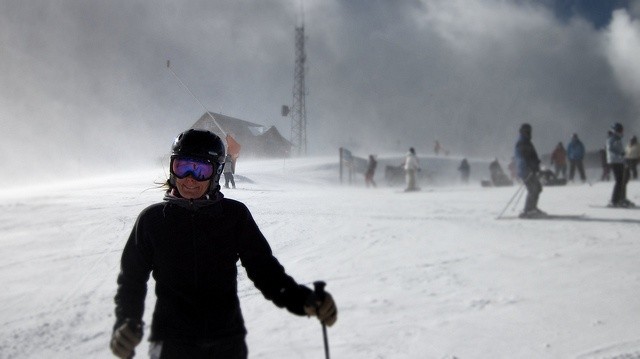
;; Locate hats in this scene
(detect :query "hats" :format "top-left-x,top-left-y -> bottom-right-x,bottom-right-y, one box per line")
520,125 -> 530,133
611,122 -> 624,132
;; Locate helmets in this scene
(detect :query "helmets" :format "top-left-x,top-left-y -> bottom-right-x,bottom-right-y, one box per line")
172,129 -> 227,194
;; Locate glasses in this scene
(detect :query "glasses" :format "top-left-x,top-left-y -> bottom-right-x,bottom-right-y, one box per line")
170,154 -> 217,181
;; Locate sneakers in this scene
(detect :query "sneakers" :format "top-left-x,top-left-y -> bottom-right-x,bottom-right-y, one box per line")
524,207 -> 543,217
610,199 -> 629,207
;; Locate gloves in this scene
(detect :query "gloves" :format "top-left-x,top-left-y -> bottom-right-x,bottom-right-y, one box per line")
288,284 -> 337,326
111,309 -> 143,358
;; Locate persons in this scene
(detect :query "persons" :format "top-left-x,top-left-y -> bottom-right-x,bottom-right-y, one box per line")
565,131 -> 586,182
513,122 -> 547,219
508,156 -> 521,185
222,153 -> 237,190
550,141 -> 567,181
404,146 -> 420,192
458,159 -> 470,184
489,156 -> 503,187
110,128 -> 337,359
605,122 -> 635,208
599,144 -> 612,182
364,154 -> 380,188
624,136 -> 640,180
